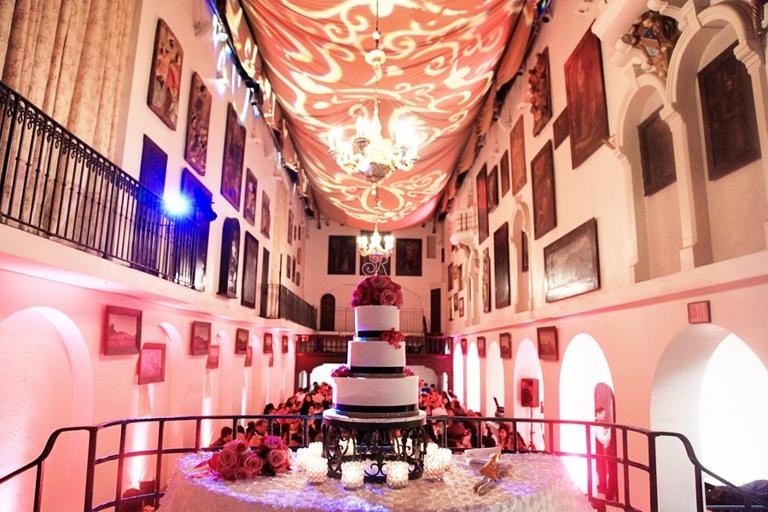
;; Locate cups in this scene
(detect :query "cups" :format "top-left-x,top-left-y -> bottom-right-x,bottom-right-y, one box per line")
386,460 -> 409,490
341,461 -> 365,490
424,456 -> 442,480
305,456 -> 328,484
297,447 -> 315,471
436,446 -> 453,471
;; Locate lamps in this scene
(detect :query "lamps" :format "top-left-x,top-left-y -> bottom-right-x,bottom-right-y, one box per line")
313,0 -> 427,201
355,220 -> 396,275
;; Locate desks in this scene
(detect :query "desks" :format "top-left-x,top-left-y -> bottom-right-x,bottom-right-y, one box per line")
157,447 -> 599,512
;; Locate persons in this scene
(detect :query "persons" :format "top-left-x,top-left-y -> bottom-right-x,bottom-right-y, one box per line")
595,406 -> 612,492
211,382 -> 333,449
419,380 -> 525,453
124,489 -> 154,512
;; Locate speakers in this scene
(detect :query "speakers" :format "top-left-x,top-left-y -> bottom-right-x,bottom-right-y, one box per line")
426,235 -> 436,259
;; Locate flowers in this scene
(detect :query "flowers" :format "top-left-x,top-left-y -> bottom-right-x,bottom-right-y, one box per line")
350,276 -> 406,306
208,434 -> 291,480
